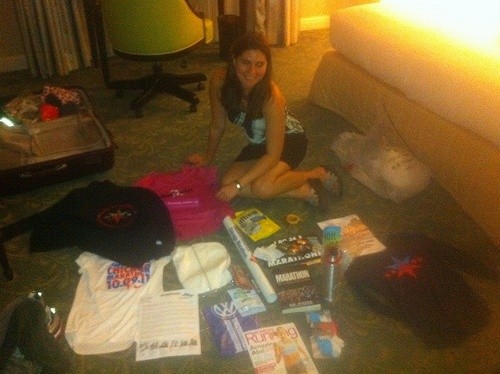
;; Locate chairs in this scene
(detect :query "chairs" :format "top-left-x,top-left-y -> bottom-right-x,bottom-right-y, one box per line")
100,0 -> 215,116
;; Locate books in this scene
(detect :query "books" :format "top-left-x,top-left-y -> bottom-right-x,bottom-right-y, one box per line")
135,290 -> 201,362
244,322 -> 321,374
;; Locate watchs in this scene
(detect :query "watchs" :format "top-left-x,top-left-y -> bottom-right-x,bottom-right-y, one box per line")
234,181 -> 241,190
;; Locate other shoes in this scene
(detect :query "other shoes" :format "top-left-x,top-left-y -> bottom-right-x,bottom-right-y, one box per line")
304,180 -> 323,210
318,163 -> 343,197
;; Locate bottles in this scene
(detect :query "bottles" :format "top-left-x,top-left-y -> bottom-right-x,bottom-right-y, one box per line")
320,248 -> 341,305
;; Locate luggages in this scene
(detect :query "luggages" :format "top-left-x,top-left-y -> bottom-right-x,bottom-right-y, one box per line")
0,85 -> 119,198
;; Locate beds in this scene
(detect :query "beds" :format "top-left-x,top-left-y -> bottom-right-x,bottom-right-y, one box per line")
307,0 -> 500,251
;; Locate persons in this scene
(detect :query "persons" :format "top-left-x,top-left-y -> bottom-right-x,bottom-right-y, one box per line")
273,327 -> 311,373
182,30 -> 341,208
